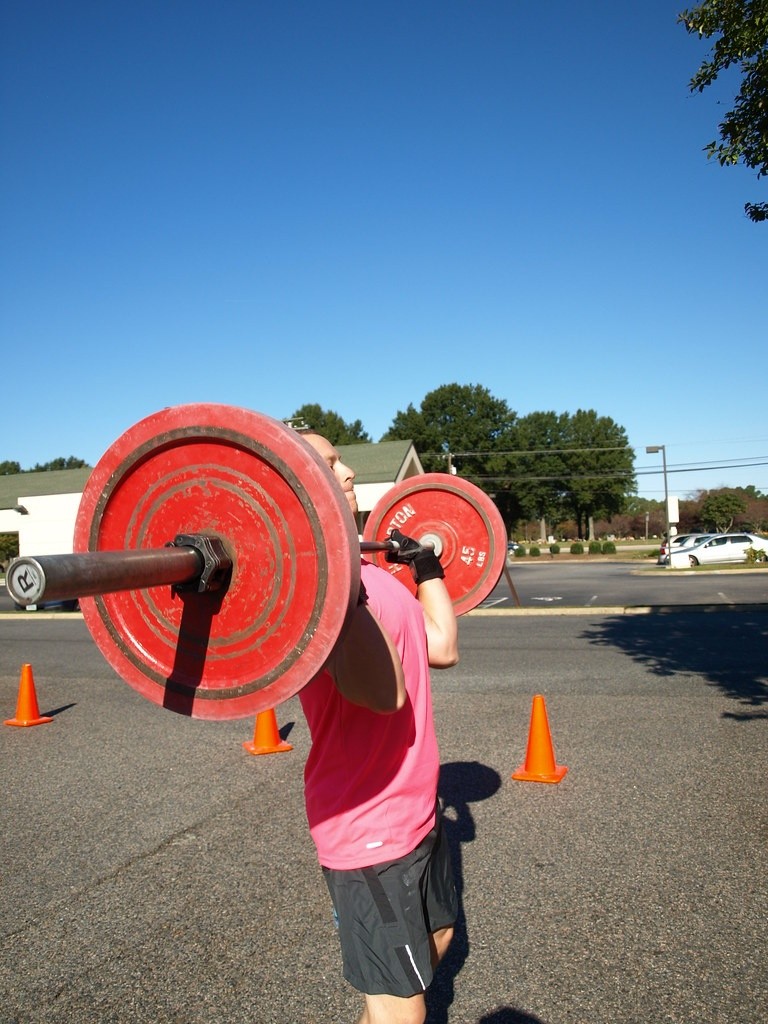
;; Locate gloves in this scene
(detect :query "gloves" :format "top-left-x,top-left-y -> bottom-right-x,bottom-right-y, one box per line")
384,529 -> 446,586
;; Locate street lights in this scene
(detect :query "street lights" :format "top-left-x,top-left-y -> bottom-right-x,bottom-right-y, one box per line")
646,444 -> 671,568
512,695 -> 567,784
3,663 -> 54,727
242,709 -> 294,755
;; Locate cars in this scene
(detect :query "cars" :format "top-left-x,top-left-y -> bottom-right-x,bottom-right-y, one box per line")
658,532 -> 768,569
506,542 -> 524,557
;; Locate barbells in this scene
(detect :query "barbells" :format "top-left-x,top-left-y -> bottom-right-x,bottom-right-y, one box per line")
6,402 -> 509,720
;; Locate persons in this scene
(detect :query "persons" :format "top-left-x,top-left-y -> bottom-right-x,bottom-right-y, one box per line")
293,429 -> 458,1024
